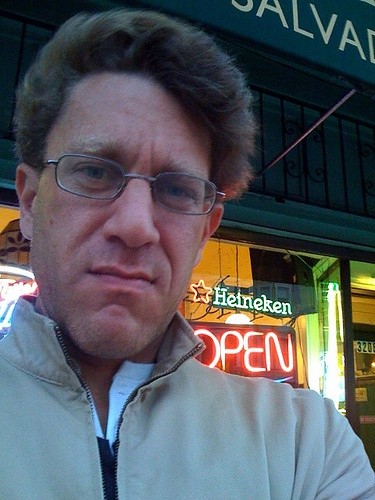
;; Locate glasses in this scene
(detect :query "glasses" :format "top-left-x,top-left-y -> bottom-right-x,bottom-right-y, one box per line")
44,153 -> 226,216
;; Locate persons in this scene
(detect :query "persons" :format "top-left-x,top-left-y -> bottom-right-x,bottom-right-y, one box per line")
0,11 -> 375,500
